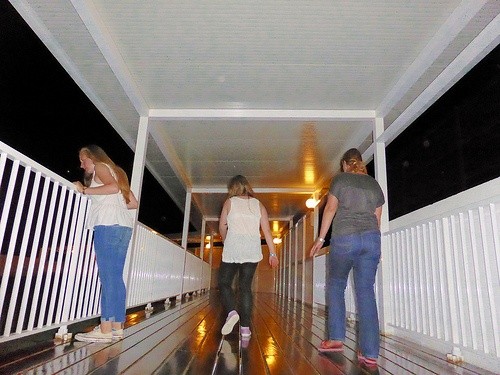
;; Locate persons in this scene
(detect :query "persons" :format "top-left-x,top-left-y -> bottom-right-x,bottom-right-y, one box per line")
219,174 -> 279,336
72,145 -> 137,342
310,147 -> 386,367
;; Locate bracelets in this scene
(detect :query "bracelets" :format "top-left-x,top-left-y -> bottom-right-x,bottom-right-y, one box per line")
318,237 -> 325,242
270,253 -> 276,256
83,186 -> 87,194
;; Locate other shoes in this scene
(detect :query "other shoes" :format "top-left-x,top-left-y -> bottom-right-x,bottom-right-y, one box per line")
221,310 -> 240,335
317,337 -> 345,353
112,328 -> 124,341
240,325 -> 252,337
357,349 -> 378,366
75,324 -> 112,342
